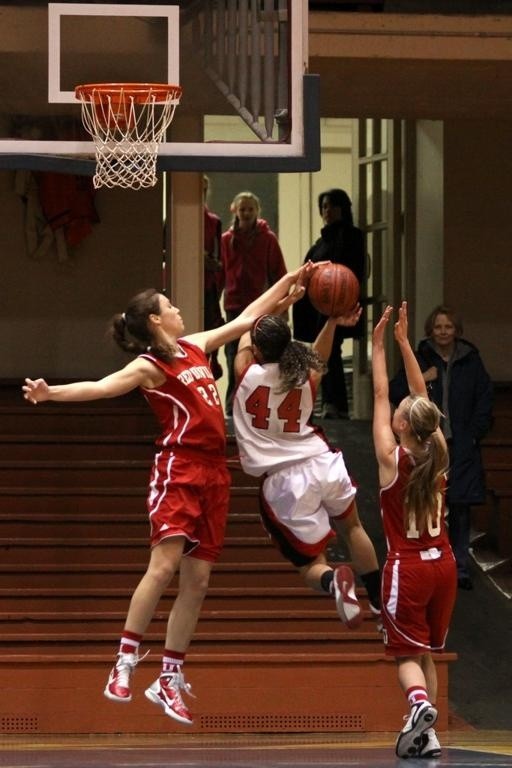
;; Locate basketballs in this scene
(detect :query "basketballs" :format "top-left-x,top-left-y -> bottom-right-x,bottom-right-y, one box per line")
307,263 -> 359,317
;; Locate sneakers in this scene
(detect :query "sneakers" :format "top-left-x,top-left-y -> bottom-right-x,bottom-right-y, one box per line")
143,671 -> 201,726
394,698 -> 438,760
416,728 -> 443,763
456,577 -> 474,592
311,403 -> 341,421
368,601 -> 389,633
332,561 -> 365,632
103,648 -> 153,705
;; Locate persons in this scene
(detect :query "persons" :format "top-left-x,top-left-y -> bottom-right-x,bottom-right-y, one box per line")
163,175 -> 223,380
219,190 -> 290,416
389,307 -> 492,588
233,258 -> 382,630
23,261 -> 331,725
292,189 -> 368,421
374,300 -> 458,760
